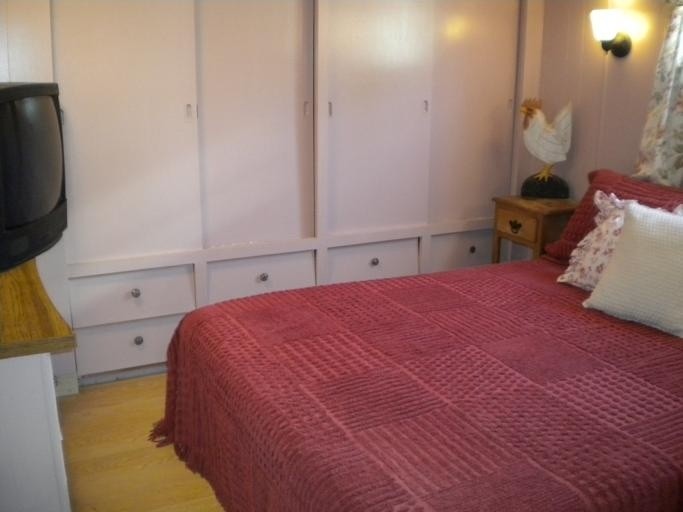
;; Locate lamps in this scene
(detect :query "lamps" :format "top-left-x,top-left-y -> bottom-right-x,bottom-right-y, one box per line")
592,8 -> 631,58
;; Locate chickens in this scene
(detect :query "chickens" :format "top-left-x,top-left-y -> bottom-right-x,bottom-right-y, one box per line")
520,94 -> 573,182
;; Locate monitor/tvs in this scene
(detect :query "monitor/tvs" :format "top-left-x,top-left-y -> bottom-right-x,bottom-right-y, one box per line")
0,81 -> 67,271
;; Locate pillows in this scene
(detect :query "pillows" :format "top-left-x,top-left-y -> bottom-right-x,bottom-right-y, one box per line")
544,168 -> 682,339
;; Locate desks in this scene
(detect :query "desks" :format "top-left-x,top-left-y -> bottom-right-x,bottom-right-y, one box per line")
1,258 -> 78,512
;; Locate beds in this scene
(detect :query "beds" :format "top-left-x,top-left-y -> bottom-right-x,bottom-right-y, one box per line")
149,255 -> 682,512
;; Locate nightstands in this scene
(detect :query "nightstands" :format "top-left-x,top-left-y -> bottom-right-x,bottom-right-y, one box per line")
491,194 -> 576,265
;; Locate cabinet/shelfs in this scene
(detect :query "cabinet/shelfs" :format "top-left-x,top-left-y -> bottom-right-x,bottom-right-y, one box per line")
62,258 -> 203,324
200,255 -> 319,307
66,317 -> 201,378
322,237 -> 427,281
2,0 -> 317,255
312,1 -> 521,242
418,224 -> 492,275
